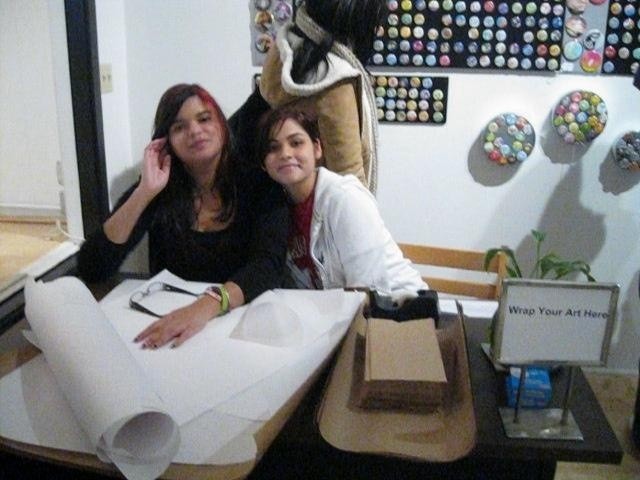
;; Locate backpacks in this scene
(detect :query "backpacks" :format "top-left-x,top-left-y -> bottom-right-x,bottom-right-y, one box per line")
221,73 -> 303,201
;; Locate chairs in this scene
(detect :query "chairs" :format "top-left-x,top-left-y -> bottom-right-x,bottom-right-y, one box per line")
394,241 -> 508,301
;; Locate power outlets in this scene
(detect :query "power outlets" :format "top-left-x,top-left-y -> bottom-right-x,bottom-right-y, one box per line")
98,62 -> 114,96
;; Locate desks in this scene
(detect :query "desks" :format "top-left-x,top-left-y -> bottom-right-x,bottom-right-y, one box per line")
1,271 -> 623,480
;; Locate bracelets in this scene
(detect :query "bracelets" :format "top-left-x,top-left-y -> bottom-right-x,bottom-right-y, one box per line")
204,289 -> 234,311
211,285 -> 229,316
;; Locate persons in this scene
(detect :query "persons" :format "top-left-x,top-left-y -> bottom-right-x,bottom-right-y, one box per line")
76,82 -> 295,350
258,0 -> 386,197
255,105 -> 430,318
